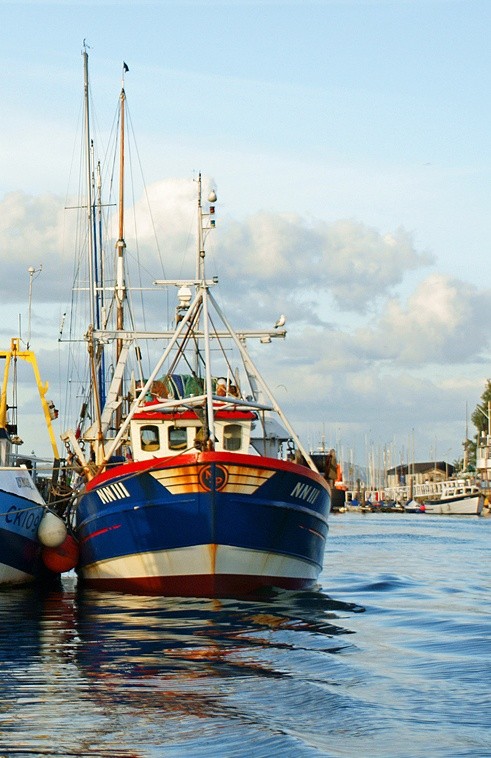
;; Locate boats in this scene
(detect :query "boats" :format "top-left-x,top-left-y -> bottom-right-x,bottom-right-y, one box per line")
0,264 -> 67,588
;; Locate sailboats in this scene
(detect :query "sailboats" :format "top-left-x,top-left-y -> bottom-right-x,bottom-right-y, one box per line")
291,400 -> 485,516
57,37 -> 334,602
